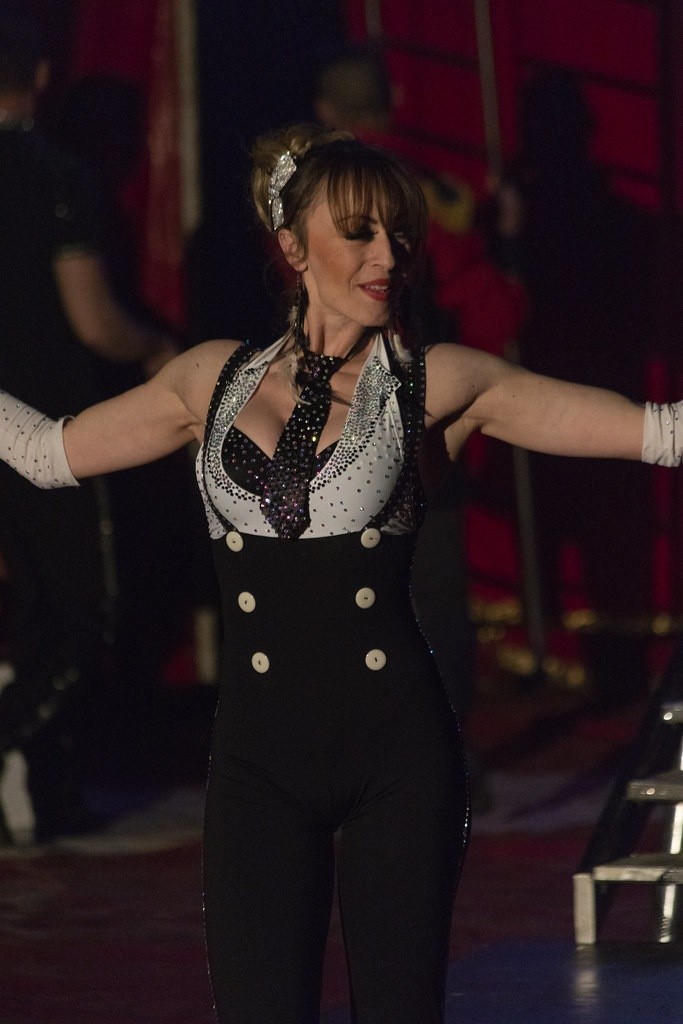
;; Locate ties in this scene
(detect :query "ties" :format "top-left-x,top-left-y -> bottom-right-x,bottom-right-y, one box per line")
260,348 -> 346,551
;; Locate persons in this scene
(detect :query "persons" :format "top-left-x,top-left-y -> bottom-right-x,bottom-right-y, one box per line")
1,125 -> 683,1024
0,0 -> 182,848
